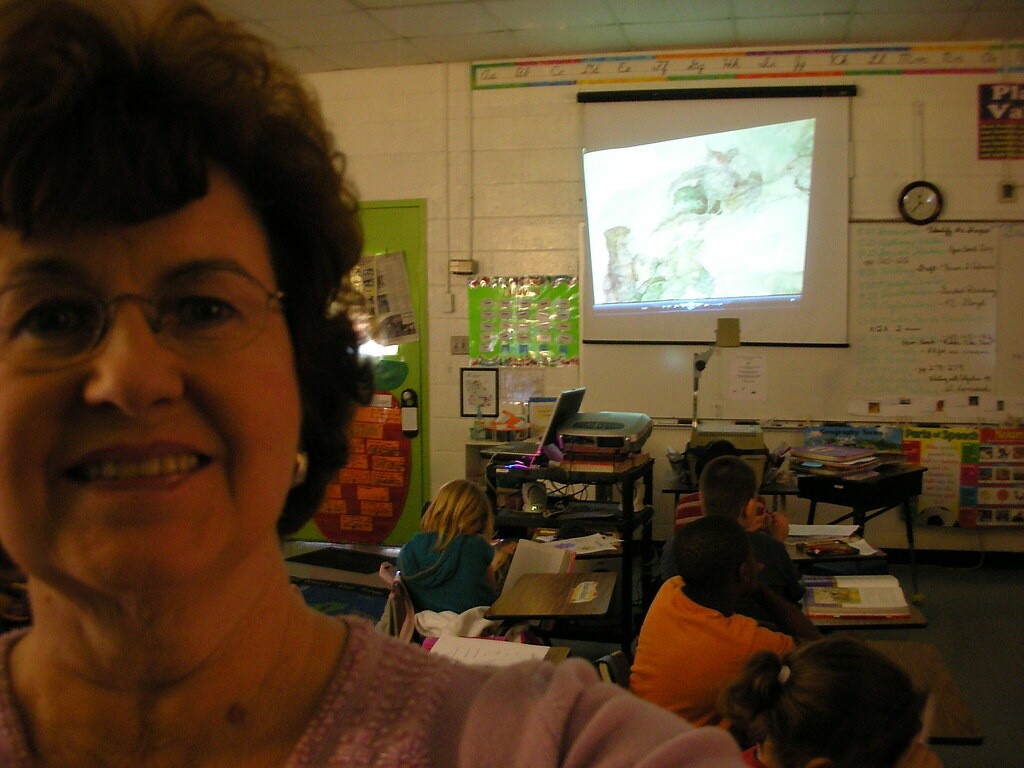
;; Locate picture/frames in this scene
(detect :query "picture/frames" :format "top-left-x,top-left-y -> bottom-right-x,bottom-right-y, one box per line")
459,367 -> 499,418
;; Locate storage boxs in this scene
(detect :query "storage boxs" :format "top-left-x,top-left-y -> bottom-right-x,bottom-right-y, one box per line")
688,425 -> 767,489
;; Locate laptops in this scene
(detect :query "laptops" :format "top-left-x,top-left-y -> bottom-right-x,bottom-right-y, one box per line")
482,387 -> 587,460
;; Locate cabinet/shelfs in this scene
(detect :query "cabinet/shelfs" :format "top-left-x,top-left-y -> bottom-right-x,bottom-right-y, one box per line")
486,458 -> 657,665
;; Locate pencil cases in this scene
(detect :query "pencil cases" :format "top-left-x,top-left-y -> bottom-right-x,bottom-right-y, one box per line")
795,539 -> 860,558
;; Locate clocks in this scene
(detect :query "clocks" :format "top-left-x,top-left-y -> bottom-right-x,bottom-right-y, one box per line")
899,180 -> 942,226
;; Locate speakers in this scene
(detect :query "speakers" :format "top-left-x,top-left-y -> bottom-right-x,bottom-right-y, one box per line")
522,481 -> 548,514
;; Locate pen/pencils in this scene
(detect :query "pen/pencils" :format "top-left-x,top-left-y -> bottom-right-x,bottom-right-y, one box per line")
811,546 -> 852,552
803,541 -> 840,546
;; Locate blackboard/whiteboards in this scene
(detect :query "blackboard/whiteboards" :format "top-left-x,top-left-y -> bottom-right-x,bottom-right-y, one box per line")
578,218 -> 1024,428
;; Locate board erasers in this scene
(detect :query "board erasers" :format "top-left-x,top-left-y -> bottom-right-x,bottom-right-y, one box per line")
917,423 -> 941,428
822,421 -> 848,428
677,419 -> 701,424
734,420 -> 759,426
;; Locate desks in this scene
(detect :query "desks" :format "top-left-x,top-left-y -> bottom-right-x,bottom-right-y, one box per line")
797,465 -> 929,605
799,591 -> 982,747
662,475 -> 801,513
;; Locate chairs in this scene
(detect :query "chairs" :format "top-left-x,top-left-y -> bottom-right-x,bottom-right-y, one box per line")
391,578 -> 407,636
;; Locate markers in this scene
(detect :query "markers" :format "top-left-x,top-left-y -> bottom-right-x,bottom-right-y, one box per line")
875,425 -> 896,428
775,424 -> 804,427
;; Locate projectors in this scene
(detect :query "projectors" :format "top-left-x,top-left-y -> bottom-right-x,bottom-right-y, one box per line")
558,411 -> 653,457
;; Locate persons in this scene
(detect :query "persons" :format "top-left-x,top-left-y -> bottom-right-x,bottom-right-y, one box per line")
658,456 -> 807,636
629,517 -> 823,729
0,1 -> 745,768
395,478 -> 496,617
717,638 -> 943,768
658,439 -> 807,635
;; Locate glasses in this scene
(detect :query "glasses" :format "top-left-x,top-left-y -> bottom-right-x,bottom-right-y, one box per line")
0,268 -> 309,376
